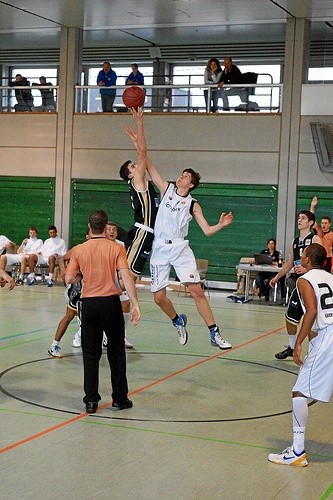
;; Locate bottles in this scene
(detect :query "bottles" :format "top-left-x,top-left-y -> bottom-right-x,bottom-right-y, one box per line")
278,254 -> 283,269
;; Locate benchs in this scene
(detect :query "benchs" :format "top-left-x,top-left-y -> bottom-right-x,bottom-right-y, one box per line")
10,263 -> 60,284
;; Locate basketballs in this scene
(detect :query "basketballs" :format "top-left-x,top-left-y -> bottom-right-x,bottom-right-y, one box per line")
122,87 -> 145,110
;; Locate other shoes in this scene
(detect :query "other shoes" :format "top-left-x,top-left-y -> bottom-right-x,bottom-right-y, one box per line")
27,278 -> 36,285
17,278 -> 23,285
48,278 -> 53,287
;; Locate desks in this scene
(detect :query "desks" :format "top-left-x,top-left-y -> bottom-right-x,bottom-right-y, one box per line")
236,264 -> 286,306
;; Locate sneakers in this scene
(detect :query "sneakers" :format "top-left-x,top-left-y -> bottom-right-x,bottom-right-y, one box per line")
124,338 -> 133,349
268,446 -> 308,467
48,345 -> 63,359
72,332 -> 82,348
275,346 -> 294,359
102,337 -> 107,348
210,327 -> 232,350
173,314 -> 188,345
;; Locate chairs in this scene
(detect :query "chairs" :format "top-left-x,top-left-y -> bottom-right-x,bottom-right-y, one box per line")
177,258 -> 211,298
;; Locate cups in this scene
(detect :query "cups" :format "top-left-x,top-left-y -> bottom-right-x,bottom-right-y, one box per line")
294,260 -> 301,272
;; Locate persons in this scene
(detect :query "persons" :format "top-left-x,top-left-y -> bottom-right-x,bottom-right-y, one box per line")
31,76 -> 54,112
10,74 -> 34,112
126,64 -> 144,85
204,58 -> 222,107
310,197 -> 333,274
270,210 -> 323,359
97,62 -> 117,112
120,107 -> 159,280
48,228 -> 91,358
104,223 -> 133,347
124,125 -> 233,351
268,242 -> 333,466
255,239 -> 280,301
218,57 -> 249,110
0,226 -> 66,291
65,211 -> 140,413
